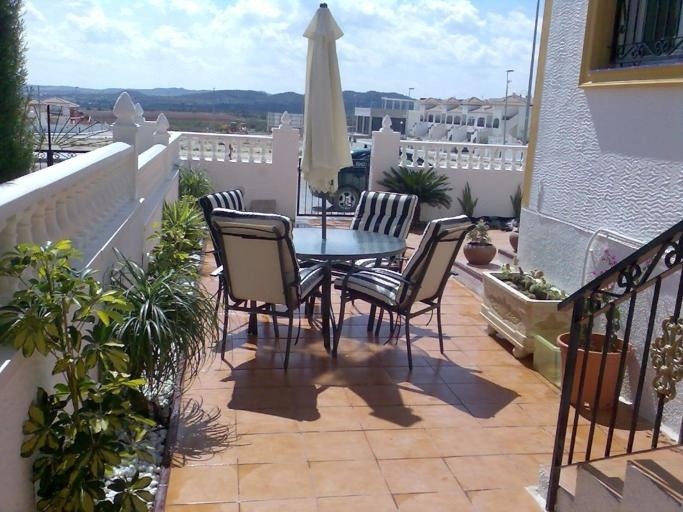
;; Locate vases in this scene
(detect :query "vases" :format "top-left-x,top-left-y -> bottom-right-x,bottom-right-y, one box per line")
556,331 -> 632,412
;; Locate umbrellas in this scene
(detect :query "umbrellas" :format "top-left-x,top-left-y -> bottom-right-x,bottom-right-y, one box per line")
297,2 -> 355,241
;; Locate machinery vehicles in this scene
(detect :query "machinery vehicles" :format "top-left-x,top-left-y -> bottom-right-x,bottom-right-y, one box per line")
223,120 -> 247,133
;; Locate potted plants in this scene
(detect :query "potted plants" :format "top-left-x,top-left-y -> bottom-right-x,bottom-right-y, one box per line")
505,219 -> 521,251
374,166 -> 452,223
479,254 -> 570,360
462,218 -> 496,264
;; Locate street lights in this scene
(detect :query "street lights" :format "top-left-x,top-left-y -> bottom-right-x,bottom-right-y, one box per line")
399,121 -> 403,135
406,86 -> 415,117
501,69 -> 516,145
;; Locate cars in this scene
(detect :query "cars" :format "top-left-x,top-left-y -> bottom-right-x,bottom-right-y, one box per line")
307,144 -> 438,211
